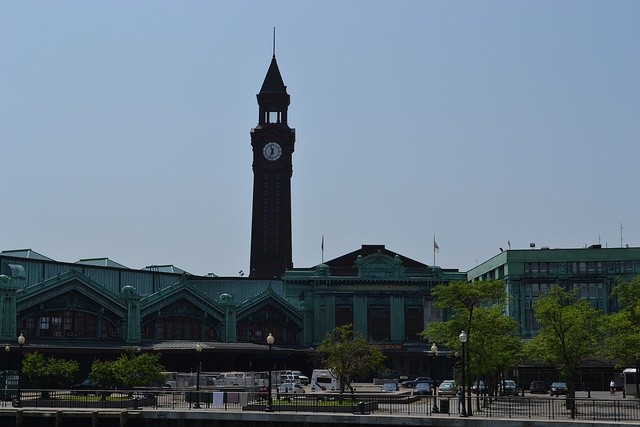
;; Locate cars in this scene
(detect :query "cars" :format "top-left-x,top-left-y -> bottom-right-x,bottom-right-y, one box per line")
382,381 -> 399,391
401,376 -> 441,387
276,367 -> 309,396
550,381 -> 570,396
470,378 -> 489,393
500,379 -> 519,395
530,380 -> 548,394
444,378 -> 458,387
413,382 -> 432,395
438,381 -> 457,396
203,369 -> 264,389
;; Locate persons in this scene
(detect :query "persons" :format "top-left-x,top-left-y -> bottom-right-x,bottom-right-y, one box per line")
609,378 -> 616,396
458,388 -> 463,401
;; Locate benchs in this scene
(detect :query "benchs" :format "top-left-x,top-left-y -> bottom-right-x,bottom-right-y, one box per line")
310,340 -> 322,348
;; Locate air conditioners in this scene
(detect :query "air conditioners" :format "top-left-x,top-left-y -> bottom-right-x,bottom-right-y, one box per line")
111,331 -> 120,337
53,332 -> 61,337
19,329 -> 27,336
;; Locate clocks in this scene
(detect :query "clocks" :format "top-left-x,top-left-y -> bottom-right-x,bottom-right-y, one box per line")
262,142 -> 282,161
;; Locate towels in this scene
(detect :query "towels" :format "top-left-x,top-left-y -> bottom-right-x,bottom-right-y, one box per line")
226,392 -> 239,403
239,392 -> 248,408
212,392 -> 224,406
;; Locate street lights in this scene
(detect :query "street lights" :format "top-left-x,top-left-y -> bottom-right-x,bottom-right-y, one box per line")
195,342 -> 202,408
2,344 -> 12,406
14,333 -> 26,407
458,329 -> 468,417
264,330 -> 275,412
430,342 -> 439,412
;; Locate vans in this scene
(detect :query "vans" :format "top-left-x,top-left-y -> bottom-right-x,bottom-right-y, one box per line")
310,368 -> 343,392
